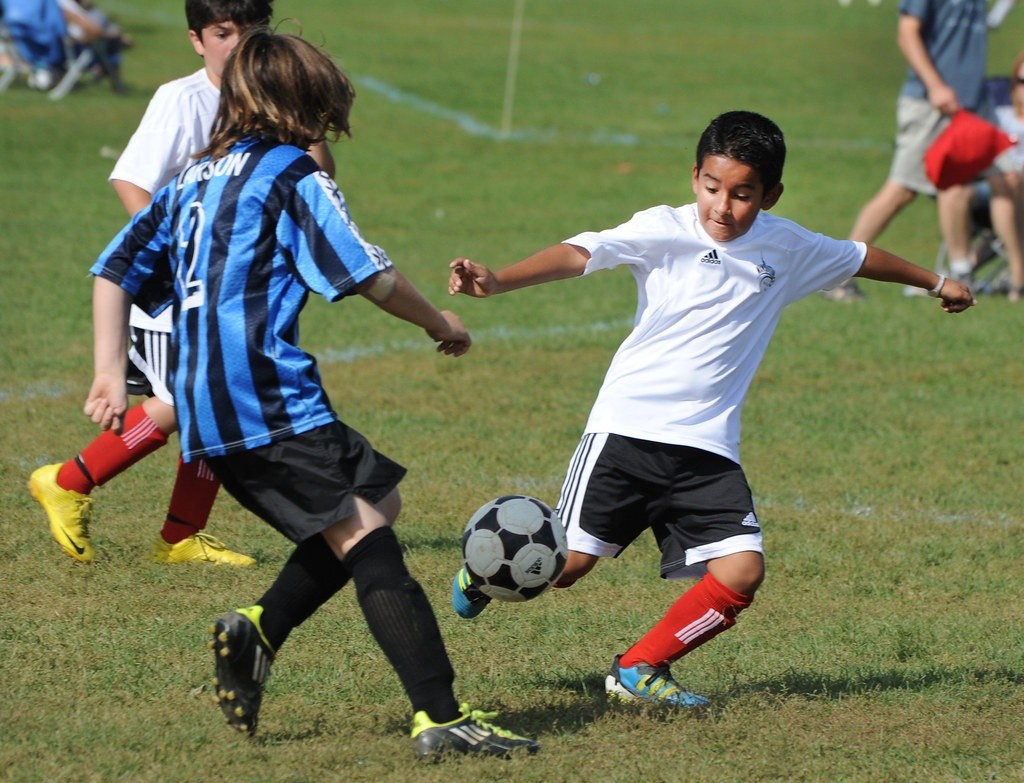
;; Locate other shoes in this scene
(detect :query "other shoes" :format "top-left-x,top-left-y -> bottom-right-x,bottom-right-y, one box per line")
821,279 -> 864,302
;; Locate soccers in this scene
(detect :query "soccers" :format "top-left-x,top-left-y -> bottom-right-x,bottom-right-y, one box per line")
457,494 -> 569,604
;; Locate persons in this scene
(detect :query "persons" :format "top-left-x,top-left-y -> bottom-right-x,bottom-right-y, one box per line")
29,0 -> 335,570
84,18 -> 542,766
448,112 -> 976,709
820,0 -> 993,303
939,56 -> 1024,294
65,1 -> 129,43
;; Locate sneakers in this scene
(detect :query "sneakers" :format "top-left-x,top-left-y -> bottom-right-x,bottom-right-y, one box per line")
152,530 -> 257,567
605,654 -> 709,708
209,606 -> 275,738
452,565 -> 492,618
27,463 -> 95,564
411,703 -> 542,761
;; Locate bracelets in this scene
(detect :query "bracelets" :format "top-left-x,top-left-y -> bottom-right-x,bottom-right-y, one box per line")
928,273 -> 946,297
